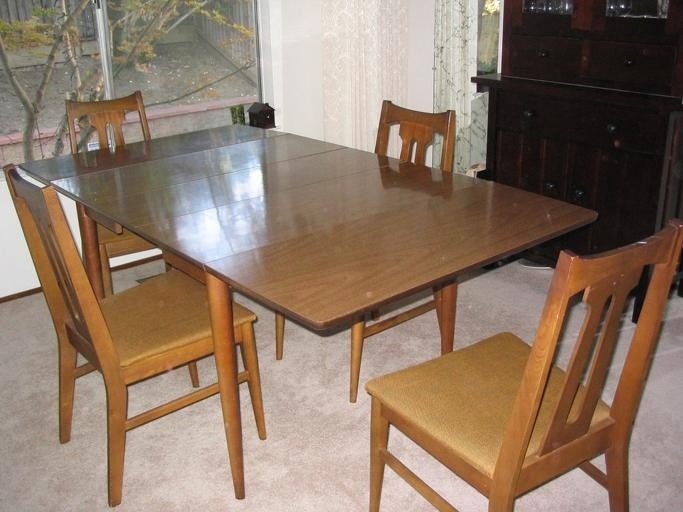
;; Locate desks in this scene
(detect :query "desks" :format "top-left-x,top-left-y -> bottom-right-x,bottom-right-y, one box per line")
18,122 -> 599,501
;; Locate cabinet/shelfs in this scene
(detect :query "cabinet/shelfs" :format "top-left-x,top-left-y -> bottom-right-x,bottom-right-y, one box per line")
467,0 -> 683,323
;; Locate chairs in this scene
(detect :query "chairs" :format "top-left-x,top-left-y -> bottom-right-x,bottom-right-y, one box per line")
363,216 -> 683,512
274,100 -> 458,404
64,91 -> 152,298
3,161 -> 267,508
